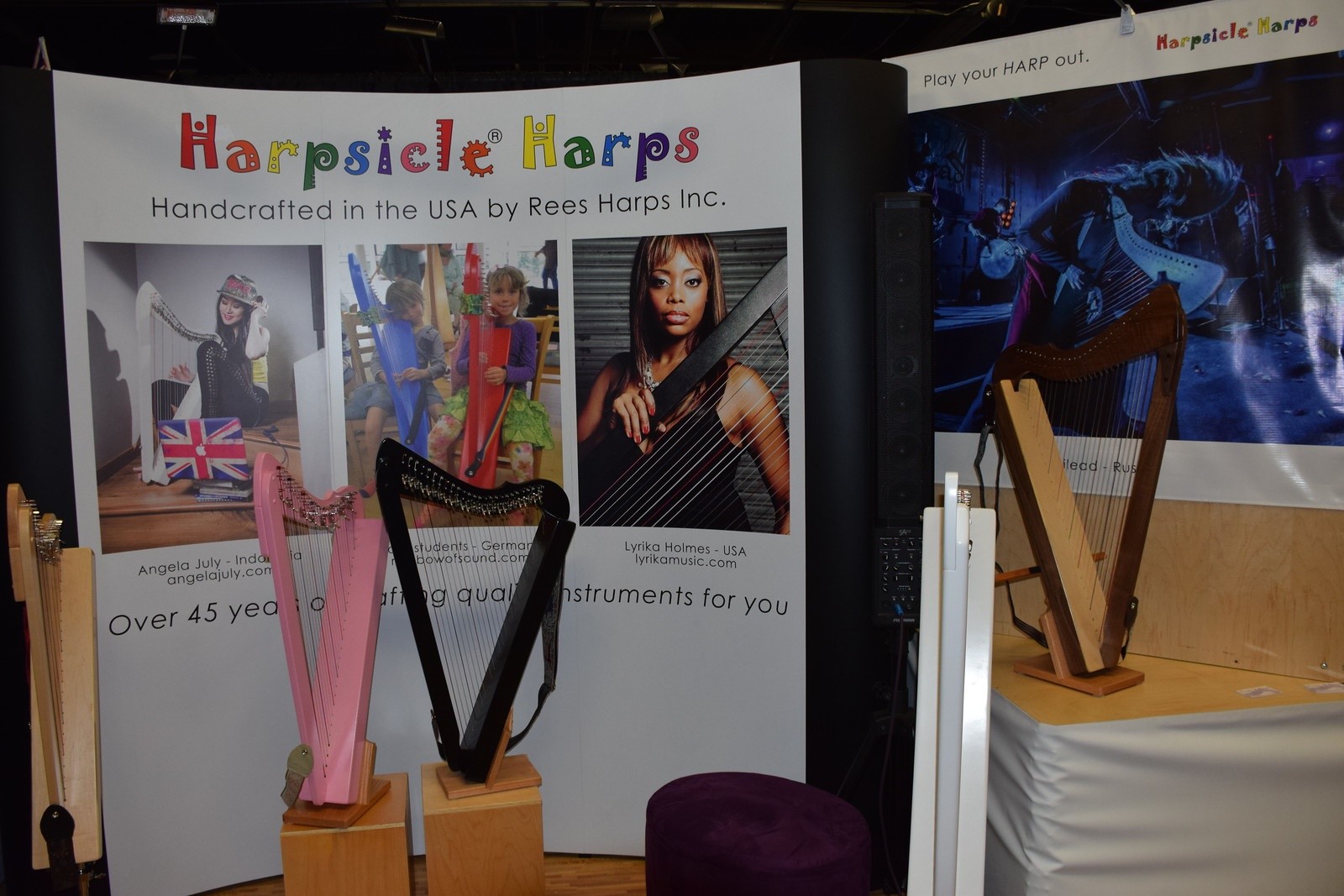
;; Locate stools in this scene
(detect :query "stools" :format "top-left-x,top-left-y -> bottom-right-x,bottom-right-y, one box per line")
642,771 -> 870,896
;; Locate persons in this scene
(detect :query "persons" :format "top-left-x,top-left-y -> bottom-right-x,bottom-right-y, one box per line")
956,154 -> 1240,433
1234,189 -> 1261,268
379,244 -> 467,334
572,232 -> 791,536
429,264 -> 536,483
967,198 -> 1010,272
359,279 -> 447,499
167,273 -> 271,429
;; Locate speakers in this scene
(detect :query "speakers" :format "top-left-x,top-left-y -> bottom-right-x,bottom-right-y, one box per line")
872,192 -> 933,627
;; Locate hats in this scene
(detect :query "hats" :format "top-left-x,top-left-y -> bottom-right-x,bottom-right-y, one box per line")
216,274 -> 258,305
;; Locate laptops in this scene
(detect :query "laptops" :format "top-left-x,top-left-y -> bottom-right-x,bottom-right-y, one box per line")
158,417 -> 254,481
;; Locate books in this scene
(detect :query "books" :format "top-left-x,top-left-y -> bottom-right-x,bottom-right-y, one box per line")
192,479 -> 253,503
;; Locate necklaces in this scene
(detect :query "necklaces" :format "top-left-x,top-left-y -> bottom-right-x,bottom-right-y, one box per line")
639,353 -> 666,392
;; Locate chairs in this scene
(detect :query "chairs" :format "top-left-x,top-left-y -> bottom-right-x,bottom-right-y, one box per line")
449,312 -> 559,480
339,308 -> 434,483
